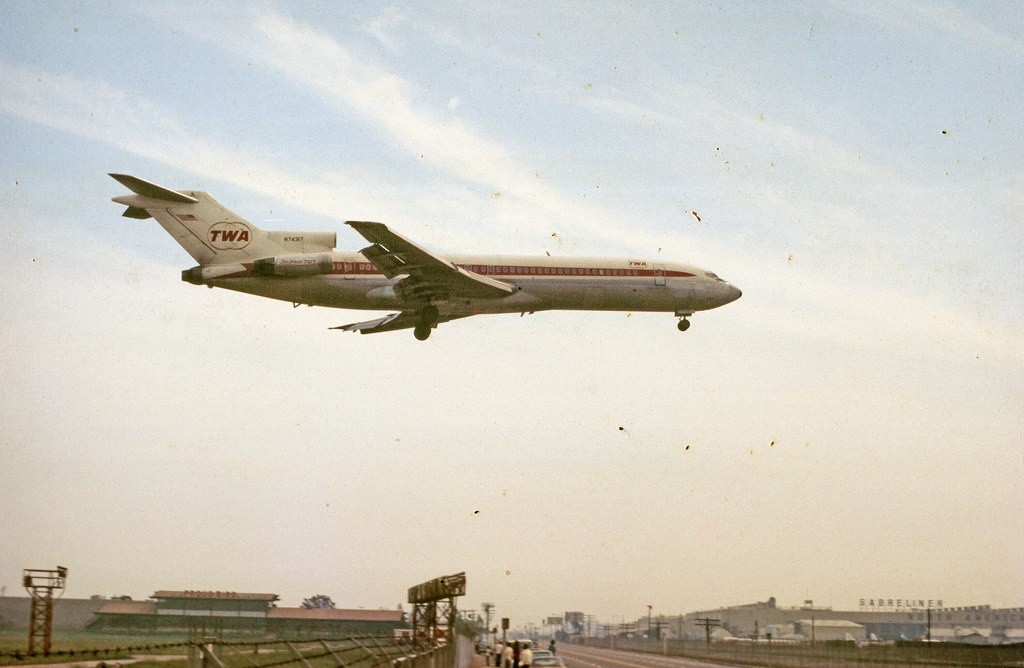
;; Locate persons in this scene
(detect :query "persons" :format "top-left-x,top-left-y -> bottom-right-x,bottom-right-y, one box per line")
200,644 -> 212,668
486,640 -> 533,668
548,639 -> 557,656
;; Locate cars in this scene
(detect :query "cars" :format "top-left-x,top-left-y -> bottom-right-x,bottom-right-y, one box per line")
478,638 -> 565,668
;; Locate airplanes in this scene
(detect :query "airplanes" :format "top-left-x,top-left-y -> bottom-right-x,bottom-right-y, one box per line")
108,172 -> 742,342
